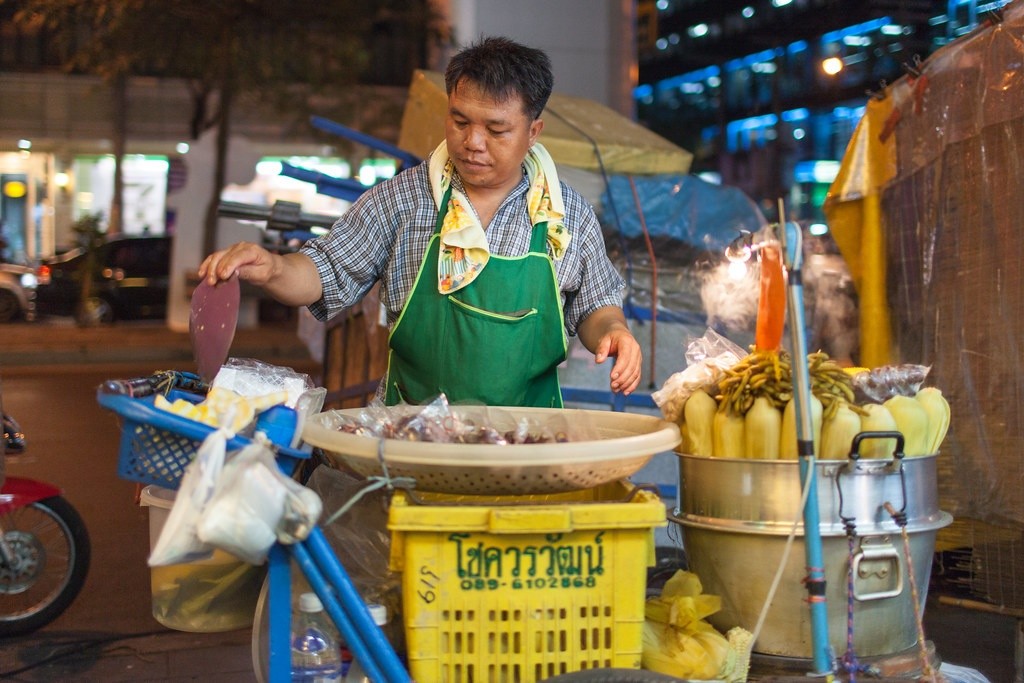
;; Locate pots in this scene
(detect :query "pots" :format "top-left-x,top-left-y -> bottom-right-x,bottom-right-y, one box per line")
667,431 -> 953,657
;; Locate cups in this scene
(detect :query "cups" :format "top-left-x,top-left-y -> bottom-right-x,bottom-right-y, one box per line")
291,593 -> 340,683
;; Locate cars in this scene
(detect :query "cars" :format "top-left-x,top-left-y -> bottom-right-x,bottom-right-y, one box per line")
36,231 -> 170,326
0,262 -> 39,327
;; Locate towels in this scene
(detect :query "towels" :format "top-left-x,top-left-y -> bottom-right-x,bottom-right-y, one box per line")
428,137 -> 572,295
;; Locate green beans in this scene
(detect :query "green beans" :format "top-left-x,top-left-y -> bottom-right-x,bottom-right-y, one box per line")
708,344 -> 869,419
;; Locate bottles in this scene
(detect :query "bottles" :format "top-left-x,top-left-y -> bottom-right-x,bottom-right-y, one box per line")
345,604 -> 387,683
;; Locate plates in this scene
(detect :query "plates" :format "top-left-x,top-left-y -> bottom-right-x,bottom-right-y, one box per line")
189,270 -> 241,383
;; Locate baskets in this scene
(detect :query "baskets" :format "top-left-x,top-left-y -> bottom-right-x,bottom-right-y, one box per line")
96,379 -> 313,491
303,405 -> 683,497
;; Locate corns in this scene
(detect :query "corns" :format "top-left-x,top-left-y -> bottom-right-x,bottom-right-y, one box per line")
675,384 -> 952,460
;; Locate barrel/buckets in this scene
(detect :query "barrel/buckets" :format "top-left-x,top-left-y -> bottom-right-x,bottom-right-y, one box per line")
139,485 -> 267,633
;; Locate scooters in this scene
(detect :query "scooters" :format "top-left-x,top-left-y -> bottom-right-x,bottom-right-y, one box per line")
0,412 -> 94,640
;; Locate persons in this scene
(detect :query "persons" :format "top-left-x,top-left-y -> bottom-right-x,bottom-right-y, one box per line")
199,37 -> 642,407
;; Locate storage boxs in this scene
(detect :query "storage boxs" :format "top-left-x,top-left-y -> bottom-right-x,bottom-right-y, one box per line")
387,479 -> 668,683
394,69 -> 694,173
97,371 -> 314,493
